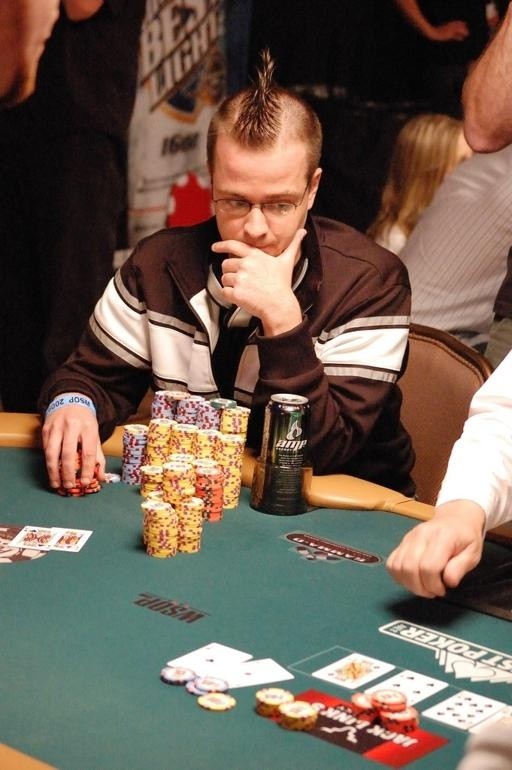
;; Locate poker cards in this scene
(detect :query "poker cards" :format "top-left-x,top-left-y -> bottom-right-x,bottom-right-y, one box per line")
167,641 -> 253,681
365,670 -> 449,708
311,652 -> 395,690
421,689 -> 512,734
228,657 -> 295,689
10,525 -> 93,552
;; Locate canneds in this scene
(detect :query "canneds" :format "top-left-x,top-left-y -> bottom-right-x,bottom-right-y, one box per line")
254,393 -> 311,511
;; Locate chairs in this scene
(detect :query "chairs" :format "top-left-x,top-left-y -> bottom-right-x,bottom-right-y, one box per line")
383,322 -> 495,504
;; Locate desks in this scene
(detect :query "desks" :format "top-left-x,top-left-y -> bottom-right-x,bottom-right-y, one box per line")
2,411 -> 511,770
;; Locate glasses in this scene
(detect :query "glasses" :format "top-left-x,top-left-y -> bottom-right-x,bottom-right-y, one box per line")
208,174 -> 311,217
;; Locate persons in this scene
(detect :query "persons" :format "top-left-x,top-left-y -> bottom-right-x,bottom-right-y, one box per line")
363,2 -> 511,373
37,49 -> 421,500
458,717 -> 511,770
1,1 -> 146,415
385,350 -> 511,599
